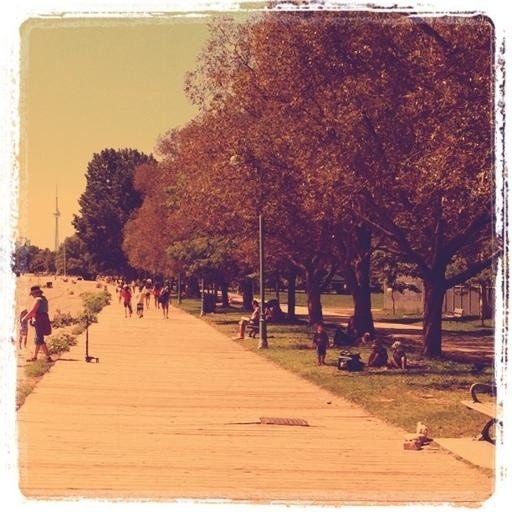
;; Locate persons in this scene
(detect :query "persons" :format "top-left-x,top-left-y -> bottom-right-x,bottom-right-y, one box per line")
311,316 -> 408,371
20,284 -> 55,362
232,300 -> 274,340
19,309 -> 30,349
108,274 -> 193,320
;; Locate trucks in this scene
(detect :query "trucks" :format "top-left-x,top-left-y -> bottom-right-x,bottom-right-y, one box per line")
279,250 -> 389,293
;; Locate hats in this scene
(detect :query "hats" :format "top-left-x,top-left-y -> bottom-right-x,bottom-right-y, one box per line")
30,287 -> 43,295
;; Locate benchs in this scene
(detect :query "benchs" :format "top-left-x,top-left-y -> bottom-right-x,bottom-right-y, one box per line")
460,383 -> 497,441
247,307 -> 273,338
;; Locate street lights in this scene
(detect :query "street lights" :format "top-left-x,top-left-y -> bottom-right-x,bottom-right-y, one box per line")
226,155 -> 269,349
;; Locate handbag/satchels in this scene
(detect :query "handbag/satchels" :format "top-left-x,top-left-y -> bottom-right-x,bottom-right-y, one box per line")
158,294 -> 166,302
35,313 -> 51,334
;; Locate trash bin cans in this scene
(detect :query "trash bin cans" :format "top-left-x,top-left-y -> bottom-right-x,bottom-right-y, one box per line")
203,295 -> 216,313
